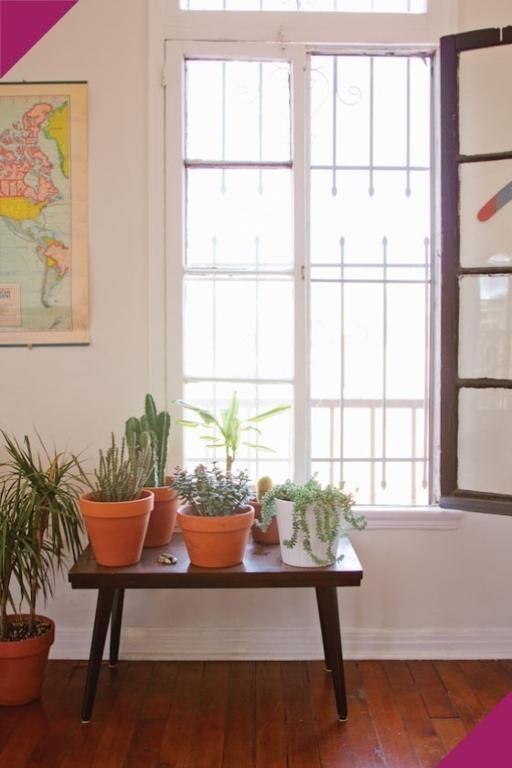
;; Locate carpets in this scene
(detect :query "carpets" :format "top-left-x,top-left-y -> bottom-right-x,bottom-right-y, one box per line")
434,692 -> 512,768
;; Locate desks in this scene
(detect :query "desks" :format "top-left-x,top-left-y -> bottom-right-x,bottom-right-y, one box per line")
67,535 -> 364,722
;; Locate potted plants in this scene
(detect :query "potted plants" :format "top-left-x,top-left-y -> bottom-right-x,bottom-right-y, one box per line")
70,433 -> 157,567
173,465 -> 253,569
124,392 -> 179,547
1,425 -> 89,706
246,476 -> 287,546
252,474 -> 365,568
